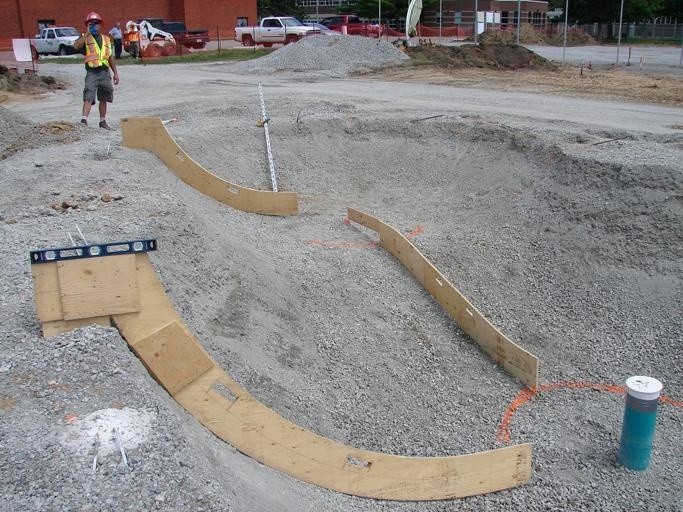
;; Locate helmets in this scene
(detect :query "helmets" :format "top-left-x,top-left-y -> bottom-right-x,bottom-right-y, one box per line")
129,23 -> 136,28
85,11 -> 103,23
116,22 -> 122,26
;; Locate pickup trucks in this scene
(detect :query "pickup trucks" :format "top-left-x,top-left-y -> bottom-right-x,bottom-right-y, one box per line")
319,15 -> 384,38
234,17 -> 320,47
29,26 -> 81,58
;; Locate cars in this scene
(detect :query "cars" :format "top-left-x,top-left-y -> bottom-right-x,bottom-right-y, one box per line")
304,22 -> 329,32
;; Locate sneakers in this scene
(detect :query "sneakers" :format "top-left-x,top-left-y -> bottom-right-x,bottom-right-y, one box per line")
81,119 -> 88,127
99,120 -> 116,131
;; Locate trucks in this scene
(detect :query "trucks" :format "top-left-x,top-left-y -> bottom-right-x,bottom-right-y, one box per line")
135,18 -> 210,51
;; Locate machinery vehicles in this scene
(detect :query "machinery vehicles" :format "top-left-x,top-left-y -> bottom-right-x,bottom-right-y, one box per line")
121,20 -> 177,58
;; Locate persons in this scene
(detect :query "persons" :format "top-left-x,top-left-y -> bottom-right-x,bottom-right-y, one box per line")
110,22 -> 123,59
128,24 -> 140,60
74,12 -> 119,129
